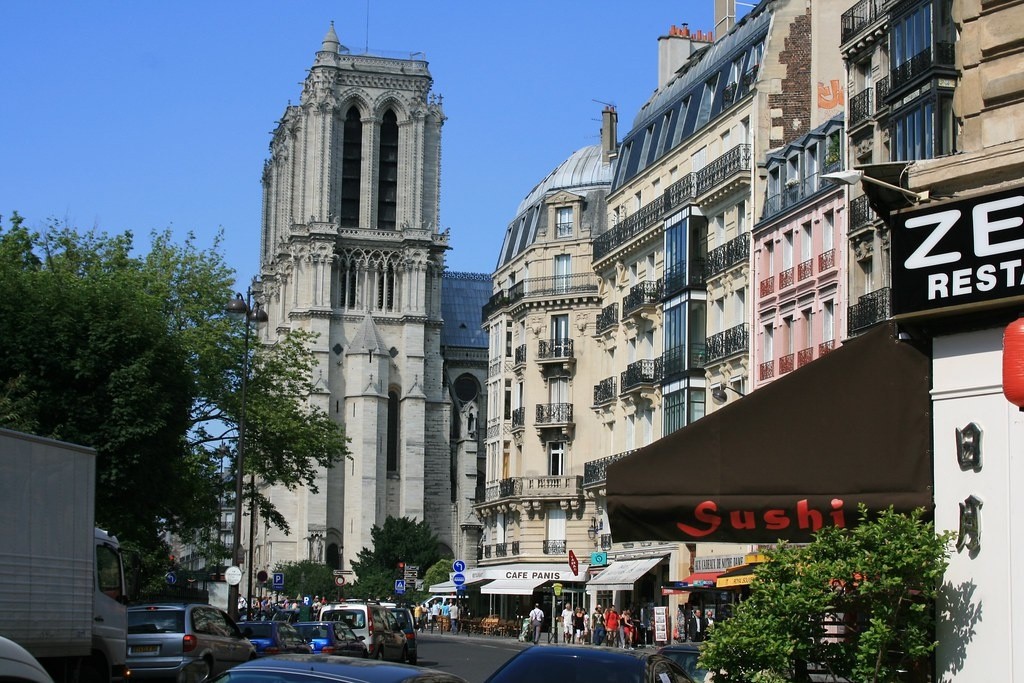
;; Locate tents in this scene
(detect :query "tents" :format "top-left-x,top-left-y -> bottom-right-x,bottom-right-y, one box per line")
716,563 -> 761,592
674,572 -> 724,590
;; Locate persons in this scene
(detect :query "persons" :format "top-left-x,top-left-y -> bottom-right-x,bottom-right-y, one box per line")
688,610 -> 704,643
561,603 -> 654,649
704,611 -> 715,641
413,599 -> 462,636
529,603 -> 544,645
237,594 -> 321,622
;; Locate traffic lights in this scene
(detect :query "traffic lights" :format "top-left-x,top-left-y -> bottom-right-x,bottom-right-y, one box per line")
170,555 -> 174,568
398,562 -> 406,582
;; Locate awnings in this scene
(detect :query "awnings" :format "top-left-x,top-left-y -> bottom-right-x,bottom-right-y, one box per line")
607,320 -> 932,543
480,579 -> 550,595
585,553 -> 670,590
428,578 -> 485,593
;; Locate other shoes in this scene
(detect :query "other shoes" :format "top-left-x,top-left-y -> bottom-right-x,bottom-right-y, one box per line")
629,647 -> 634,650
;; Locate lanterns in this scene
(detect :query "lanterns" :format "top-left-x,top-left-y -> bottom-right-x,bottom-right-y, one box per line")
1002,318 -> 1024,412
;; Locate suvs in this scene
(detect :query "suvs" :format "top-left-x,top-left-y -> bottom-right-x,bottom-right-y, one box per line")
233,619 -> 313,656
126,602 -> 257,676
290,620 -> 370,658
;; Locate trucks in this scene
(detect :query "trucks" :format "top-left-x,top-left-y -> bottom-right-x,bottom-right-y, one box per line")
0,425 -> 145,683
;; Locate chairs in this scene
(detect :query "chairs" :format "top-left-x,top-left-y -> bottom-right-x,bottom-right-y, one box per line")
436,613 -> 522,638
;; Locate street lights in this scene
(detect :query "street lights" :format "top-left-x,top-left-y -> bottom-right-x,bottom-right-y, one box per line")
551,583 -> 564,644
214,437 -> 227,581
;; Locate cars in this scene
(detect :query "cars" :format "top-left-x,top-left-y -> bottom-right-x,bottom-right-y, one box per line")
198,652 -> 469,683
654,641 -> 711,678
481,641 -> 696,683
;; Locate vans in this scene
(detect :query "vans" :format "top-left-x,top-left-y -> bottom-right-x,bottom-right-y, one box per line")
317,598 -> 410,663
417,594 -> 469,622
389,608 -> 418,663
